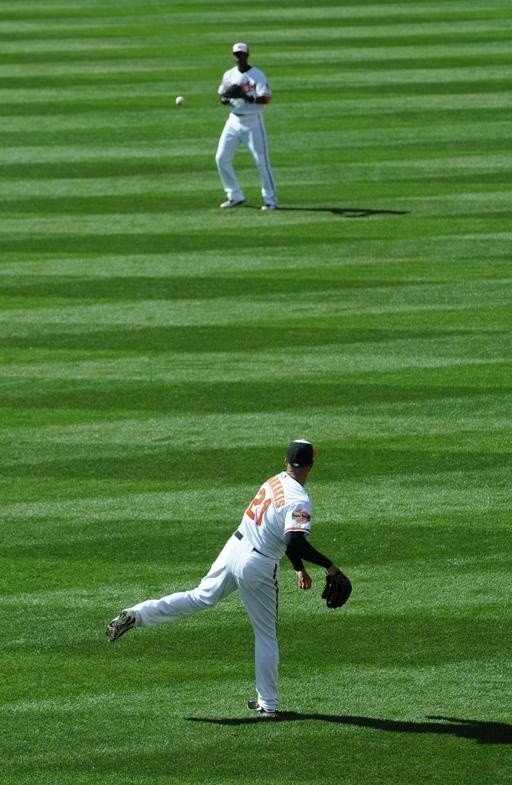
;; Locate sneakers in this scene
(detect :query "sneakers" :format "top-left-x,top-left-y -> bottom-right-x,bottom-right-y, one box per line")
101,607 -> 137,643
218,200 -> 246,209
246,699 -> 277,720
260,203 -> 278,212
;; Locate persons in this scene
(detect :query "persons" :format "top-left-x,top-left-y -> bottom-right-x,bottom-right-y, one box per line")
213,41 -> 279,213
102,436 -> 353,720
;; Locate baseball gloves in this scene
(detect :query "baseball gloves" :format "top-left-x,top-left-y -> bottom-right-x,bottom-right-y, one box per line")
224,84 -> 247,99
322,566 -> 352,609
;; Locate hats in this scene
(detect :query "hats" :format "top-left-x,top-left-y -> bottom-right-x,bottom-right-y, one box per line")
232,42 -> 249,56
286,438 -> 316,468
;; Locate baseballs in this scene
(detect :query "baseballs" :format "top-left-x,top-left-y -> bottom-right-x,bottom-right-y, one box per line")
175,96 -> 184,105
